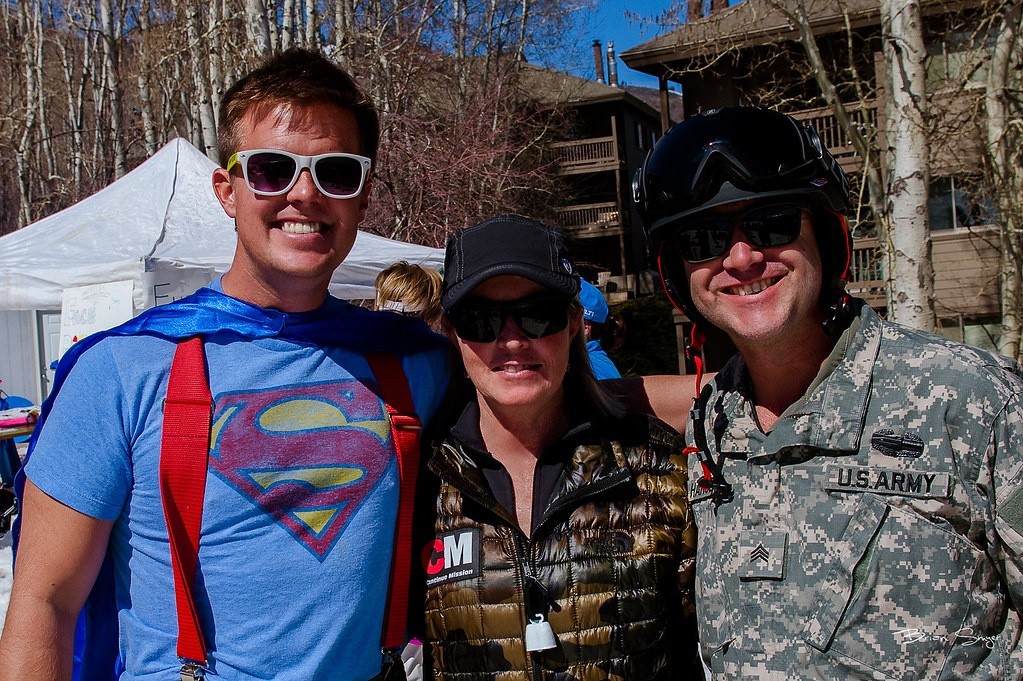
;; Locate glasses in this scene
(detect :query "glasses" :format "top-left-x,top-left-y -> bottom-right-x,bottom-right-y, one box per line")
450,288 -> 575,342
644,104 -> 808,216
669,198 -> 806,266
225,148 -> 372,199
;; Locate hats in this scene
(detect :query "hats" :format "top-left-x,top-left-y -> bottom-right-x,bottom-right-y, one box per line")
576,278 -> 609,323
438,219 -> 582,308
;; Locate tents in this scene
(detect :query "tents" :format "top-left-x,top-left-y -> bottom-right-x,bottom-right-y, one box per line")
0,136 -> 448,407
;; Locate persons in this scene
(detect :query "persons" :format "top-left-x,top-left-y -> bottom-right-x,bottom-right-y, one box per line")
375,261 -> 446,339
402,210 -> 712,681
573,270 -> 621,382
640,102 -> 1023,681
0,48 -> 720,681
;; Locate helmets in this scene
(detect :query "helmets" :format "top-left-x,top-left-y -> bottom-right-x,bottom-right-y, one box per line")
633,105 -> 852,299
